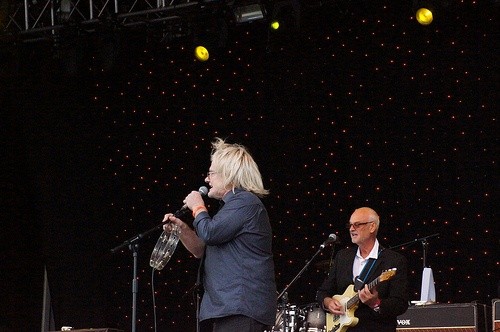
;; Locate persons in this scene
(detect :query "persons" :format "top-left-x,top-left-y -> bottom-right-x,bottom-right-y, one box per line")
317,207 -> 409,332
161,137 -> 278,332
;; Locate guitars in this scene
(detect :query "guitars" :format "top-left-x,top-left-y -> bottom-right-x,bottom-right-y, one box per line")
325,267 -> 398,332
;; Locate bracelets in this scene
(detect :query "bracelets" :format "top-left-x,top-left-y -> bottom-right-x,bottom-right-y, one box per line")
192,206 -> 207,217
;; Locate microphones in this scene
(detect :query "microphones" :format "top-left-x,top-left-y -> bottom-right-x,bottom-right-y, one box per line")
179,186 -> 208,212
320,234 -> 336,250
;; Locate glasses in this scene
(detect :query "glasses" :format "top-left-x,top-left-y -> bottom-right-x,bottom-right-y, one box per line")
207,171 -> 223,177
346,221 -> 374,229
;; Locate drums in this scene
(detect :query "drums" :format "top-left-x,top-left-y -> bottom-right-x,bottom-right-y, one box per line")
306,302 -> 326,332
